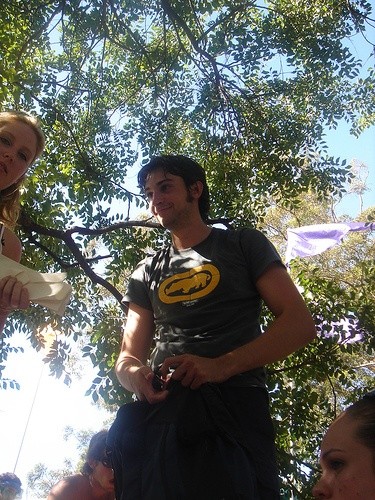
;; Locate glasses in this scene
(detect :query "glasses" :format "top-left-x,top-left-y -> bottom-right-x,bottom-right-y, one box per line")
97,456 -> 111,468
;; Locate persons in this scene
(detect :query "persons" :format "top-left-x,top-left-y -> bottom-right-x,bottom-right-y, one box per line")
0,472 -> 22,500
0,110 -> 44,332
311,388 -> 375,500
47,429 -> 115,500
108,155 -> 315,500
